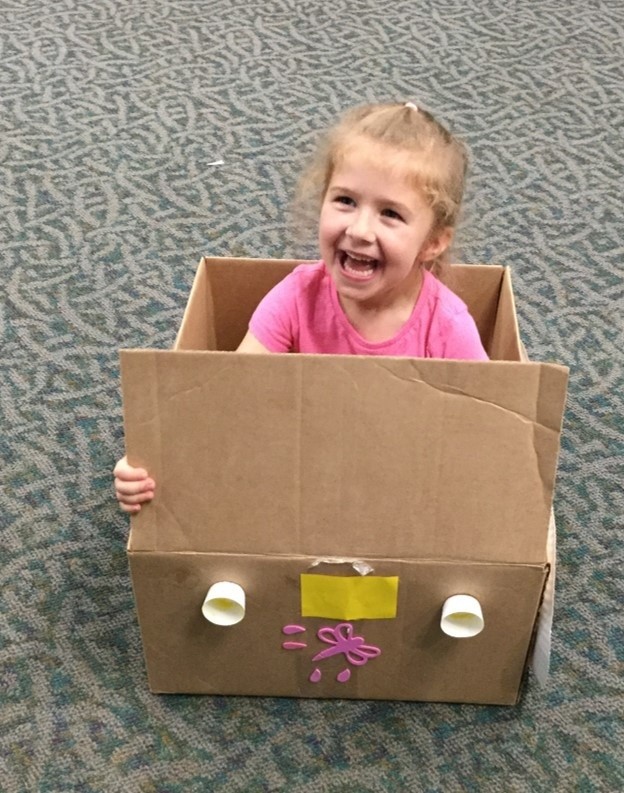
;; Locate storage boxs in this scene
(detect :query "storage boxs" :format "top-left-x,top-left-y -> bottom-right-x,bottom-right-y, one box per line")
118,257 -> 570,706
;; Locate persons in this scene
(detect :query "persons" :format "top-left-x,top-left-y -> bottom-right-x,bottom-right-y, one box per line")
114,104 -> 490,515
